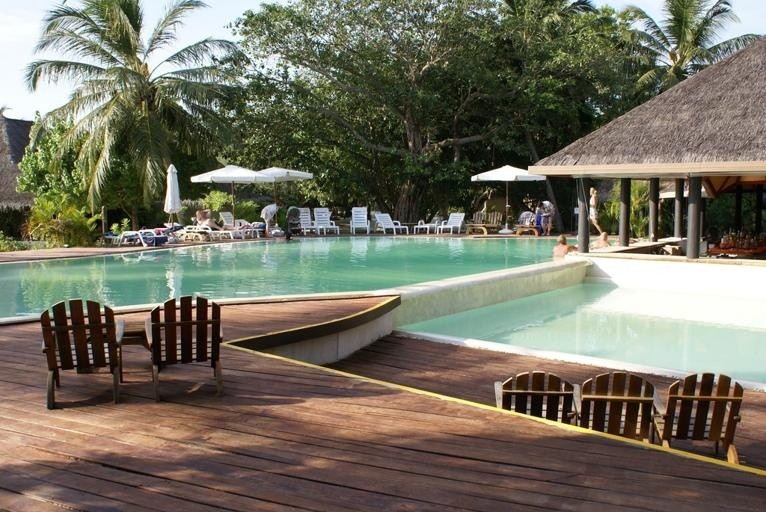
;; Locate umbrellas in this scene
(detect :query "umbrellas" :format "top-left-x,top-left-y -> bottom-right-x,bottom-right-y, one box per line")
255,167 -> 314,224
190,164 -> 273,227
470,164 -> 548,234
162,164 -> 182,227
259,201 -> 278,221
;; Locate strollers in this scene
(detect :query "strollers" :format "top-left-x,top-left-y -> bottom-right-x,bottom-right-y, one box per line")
284,206 -> 304,236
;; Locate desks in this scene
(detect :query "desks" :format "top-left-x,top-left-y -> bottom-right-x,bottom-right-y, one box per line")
73,320 -> 167,351
706,244 -> 766,259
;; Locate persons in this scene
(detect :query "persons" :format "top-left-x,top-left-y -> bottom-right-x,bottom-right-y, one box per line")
533,199 -> 555,236
553,235 -> 575,260
587,186 -> 603,232
264,219 -> 271,239
195,207 -> 236,231
585,231 -> 613,248
285,208 -> 302,237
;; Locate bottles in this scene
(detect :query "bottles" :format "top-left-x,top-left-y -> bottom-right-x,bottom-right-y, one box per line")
720,226 -> 763,250
526,213 -> 536,226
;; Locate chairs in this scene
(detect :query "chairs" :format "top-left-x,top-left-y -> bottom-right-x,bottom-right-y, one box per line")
651,372 -> 744,466
144,294 -> 224,402
494,371 -> 574,426
571,371 -> 655,441
40,299 -> 125,408
